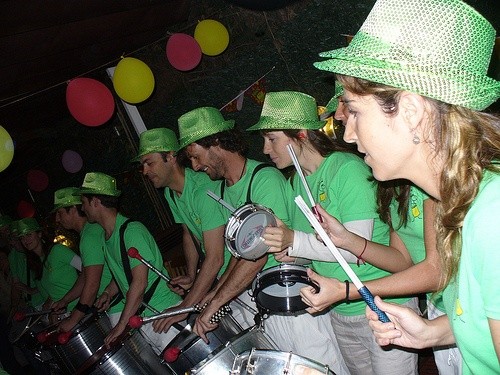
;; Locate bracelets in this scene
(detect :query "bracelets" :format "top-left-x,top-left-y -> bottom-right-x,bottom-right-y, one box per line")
63,298 -> 70,304
76,301 -> 90,314
344,280 -> 351,303
356,239 -> 368,266
103,290 -> 111,302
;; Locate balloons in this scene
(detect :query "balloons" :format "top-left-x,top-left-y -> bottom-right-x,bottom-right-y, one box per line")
27,168 -> 49,193
62,150 -> 84,174
111,55 -> 155,103
194,20 -> 230,56
17,199 -> 36,219
166,32 -> 201,71
65,78 -> 115,127
0,124 -> 14,173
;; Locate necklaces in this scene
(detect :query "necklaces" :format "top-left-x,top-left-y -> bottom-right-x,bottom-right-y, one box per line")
240,156 -> 248,182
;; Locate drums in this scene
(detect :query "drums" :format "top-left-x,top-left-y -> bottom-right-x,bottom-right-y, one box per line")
7,308 -> 114,375
228,346 -> 336,375
158,304 -> 244,375
187,324 -> 278,375
76,325 -> 176,375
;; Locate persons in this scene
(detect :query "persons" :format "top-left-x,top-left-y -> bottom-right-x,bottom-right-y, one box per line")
71,171 -> 188,351
310,1 -> 500,375
1,215 -> 50,325
242,91 -> 423,375
320,74 -> 465,374
134,125 -> 261,339
173,106 -> 350,375
46,185 -> 128,331
9,215 -> 86,318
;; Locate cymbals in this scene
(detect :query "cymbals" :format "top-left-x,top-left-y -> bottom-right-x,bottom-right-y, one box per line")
247,262 -> 322,318
222,201 -> 277,262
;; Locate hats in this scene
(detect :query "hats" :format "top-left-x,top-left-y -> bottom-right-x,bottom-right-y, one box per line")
177,107 -> 236,149
247,90 -> 328,131
312,0 -> 500,113
129,128 -> 182,163
15,217 -> 42,237
8,220 -> 21,233
72,172 -> 122,199
0,214 -> 10,227
48,187 -> 83,214
325,79 -> 345,112
